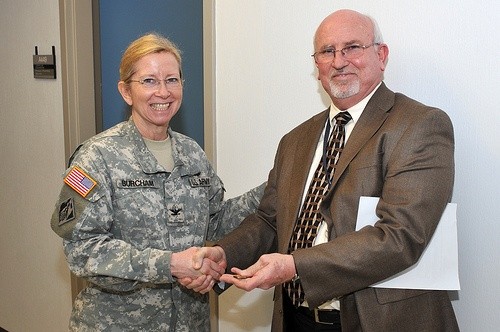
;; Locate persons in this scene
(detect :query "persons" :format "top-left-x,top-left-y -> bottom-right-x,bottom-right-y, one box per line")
178,9 -> 460,332
51,34 -> 268,332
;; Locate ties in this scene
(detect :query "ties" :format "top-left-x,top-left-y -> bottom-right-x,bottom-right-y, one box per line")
284,112 -> 352,308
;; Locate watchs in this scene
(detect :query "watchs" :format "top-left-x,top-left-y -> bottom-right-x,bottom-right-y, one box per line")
290,253 -> 300,282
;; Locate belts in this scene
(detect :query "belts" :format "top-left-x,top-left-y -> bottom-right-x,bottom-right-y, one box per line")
283,309 -> 340,326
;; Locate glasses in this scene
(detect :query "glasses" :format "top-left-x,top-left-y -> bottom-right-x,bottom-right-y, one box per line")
311,42 -> 379,64
127,76 -> 184,90
